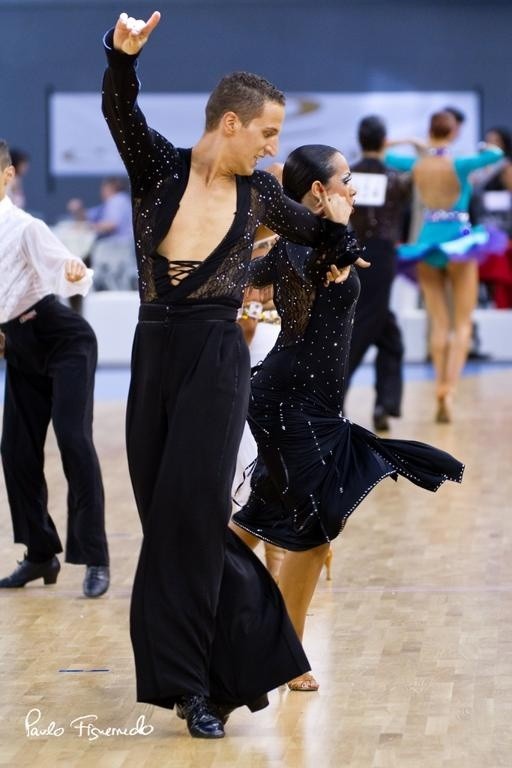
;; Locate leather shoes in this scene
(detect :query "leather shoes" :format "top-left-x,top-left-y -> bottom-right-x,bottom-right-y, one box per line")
287,674 -> 319,691
374,405 -> 390,431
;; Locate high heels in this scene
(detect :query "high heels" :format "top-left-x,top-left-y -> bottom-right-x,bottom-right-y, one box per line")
0,554 -> 60,587
176,693 -> 269,738
83,565 -> 109,597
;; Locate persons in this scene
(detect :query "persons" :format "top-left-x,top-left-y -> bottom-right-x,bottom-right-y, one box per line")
7,105 -> 511,433
99,10 -> 355,742
0,137 -> 114,598
229,146 -> 468,696
